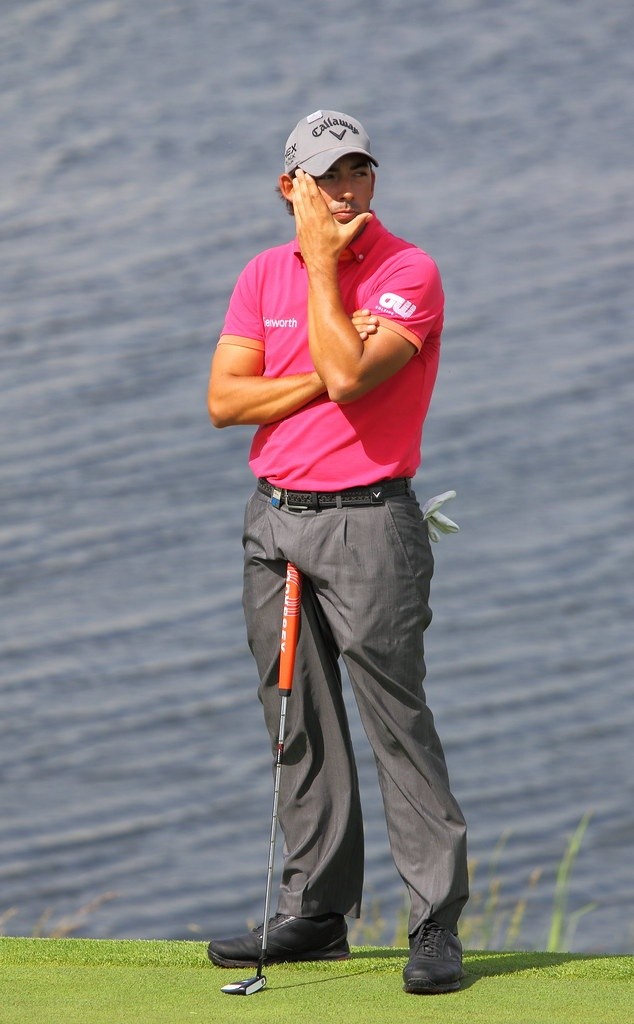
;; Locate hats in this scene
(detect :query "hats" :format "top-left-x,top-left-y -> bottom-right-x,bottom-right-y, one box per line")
284,110 -> 378,177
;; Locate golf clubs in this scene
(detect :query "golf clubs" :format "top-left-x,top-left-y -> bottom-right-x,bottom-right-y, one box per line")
220,561 -> 302,996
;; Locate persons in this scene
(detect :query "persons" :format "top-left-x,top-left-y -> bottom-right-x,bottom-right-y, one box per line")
207,110 -> 468,994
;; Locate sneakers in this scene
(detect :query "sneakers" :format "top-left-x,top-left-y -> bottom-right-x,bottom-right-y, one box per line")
404,923 -> 463,993
207,911 -> 351,966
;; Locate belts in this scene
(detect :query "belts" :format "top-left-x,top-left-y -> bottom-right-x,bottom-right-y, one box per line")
257,478 -> 412,510
